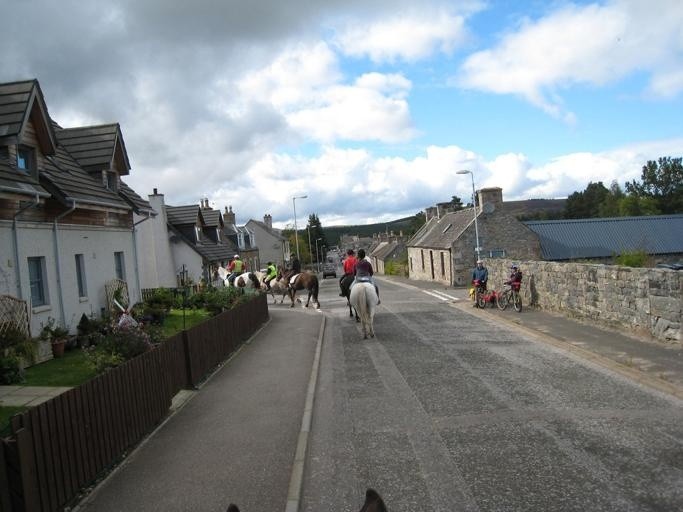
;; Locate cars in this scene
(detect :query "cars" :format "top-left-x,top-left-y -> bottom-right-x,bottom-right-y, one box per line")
322,266 -> 336,279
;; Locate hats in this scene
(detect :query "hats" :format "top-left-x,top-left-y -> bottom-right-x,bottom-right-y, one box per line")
266,261 -> 272,265
290,253 -> 296,256
511,265 -> 518,269
234,254 -> 240,259
477,260 -> 484,264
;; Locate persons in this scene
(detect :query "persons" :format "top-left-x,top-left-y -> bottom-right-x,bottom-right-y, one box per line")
500,263 -> 522,311
226,255 -> 245,289
467,259 -> 489,308
262,261 -> 276,291
336,247 -> 356,298
282,253 -> 300,289
347,247 -> 381,306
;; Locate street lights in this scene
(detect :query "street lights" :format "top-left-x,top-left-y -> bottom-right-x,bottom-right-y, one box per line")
455,170 -> 480,260
292,195 -> 307,261
307,225 -> 325,274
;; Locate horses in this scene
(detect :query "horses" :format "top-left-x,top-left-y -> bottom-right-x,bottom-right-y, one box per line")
339,274 -> 380,339
225,488 -> 387,511
209,264 -> 321,309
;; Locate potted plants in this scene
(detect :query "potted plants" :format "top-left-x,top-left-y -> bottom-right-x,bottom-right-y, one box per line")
4,317 -> 54,368
1,354 -> 25,377
48,326 -> 69,358
87,331 -> 101,346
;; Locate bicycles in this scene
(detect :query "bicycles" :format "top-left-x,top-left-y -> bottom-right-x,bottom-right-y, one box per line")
495,282 -> 521,313
469,282 -> 486,308
